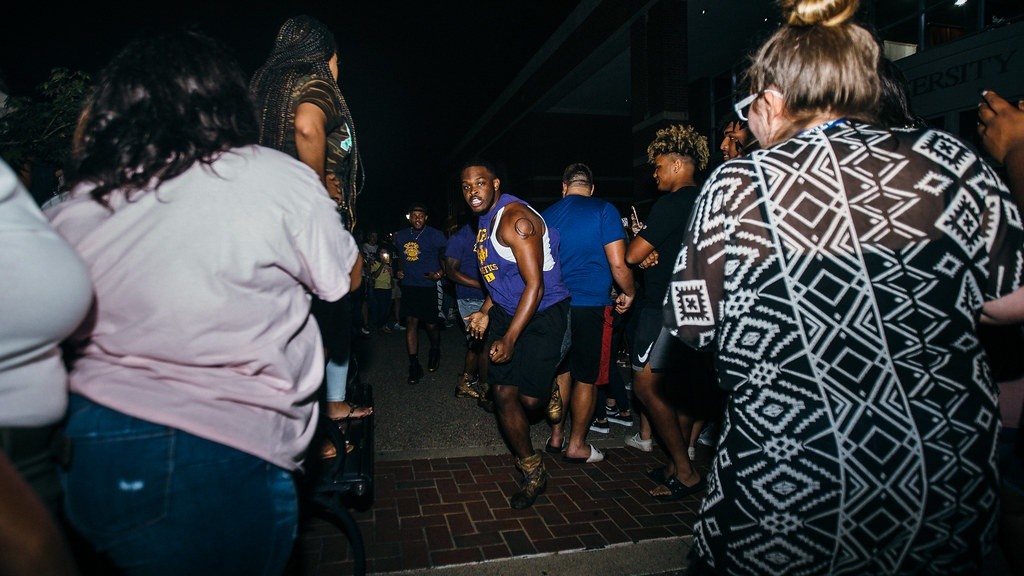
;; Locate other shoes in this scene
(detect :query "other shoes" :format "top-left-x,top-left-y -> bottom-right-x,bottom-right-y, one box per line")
392,322 -> 406,330
362,327 -> 370,334
446,323 -> 454,328
688,447 -> 703,461
383,323 -> 392,333
428,348 -> 441,372
407,364 -> 425,385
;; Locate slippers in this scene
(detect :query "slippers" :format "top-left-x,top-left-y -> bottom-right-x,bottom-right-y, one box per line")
545,436 -> 566,453
645,465 -> 677,484
322,439 -> 354,459
649,473 -> 704,500
561,444 -> 609,464
332,403 -> 375,421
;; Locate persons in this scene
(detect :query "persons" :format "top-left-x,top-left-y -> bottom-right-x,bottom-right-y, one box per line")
0,0 -> 1024,576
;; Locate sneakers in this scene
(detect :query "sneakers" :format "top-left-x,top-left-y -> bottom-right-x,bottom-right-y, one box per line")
607,411 -> 633,426
548,382 -> 562,424
625,431 -> 653,452
589,421 -> 609,434
606,405 -> 617,415
510,449 -> 547,509
480,383 -> 490,402
455,373 -> 479,398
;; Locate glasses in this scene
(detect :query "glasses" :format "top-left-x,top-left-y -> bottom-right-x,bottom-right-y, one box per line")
734,90 -> 783,121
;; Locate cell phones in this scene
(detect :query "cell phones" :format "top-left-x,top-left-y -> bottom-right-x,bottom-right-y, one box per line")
631,206 -> 640,228
